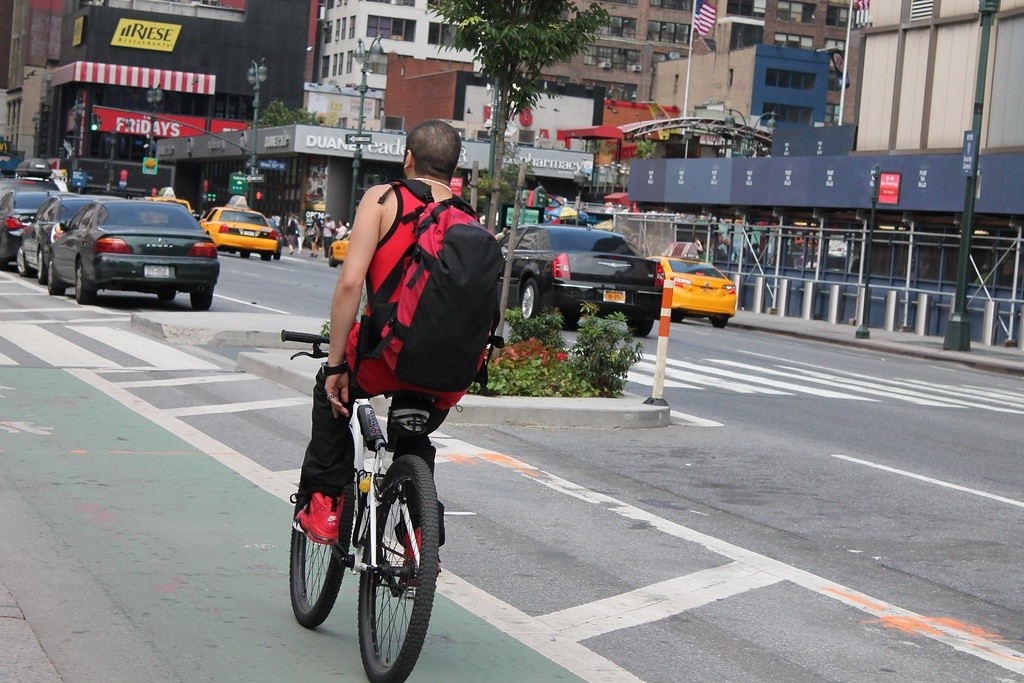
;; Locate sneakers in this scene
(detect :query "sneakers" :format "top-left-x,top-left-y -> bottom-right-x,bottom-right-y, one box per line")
295,492 -> 346,545
400,527 -> 441,587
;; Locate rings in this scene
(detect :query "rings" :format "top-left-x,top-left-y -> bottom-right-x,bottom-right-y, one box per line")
326,394 -> 334,400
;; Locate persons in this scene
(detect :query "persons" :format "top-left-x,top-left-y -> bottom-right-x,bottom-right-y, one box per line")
495,227 -> 507,242
270,214 -> 351,257
290,121 -> 506,590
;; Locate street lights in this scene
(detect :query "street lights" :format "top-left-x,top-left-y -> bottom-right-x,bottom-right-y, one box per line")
349,32 -> 384,230
724,107 -> 778,159
107,114 -> 131,190
145,84 -> 163,196
248,58 -> 268,209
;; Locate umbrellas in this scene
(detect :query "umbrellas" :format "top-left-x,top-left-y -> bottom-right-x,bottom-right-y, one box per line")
593,218 -> 631,231
548,206 -> 587,225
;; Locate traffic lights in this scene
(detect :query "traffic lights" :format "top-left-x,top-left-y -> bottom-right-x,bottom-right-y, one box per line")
91,113 -> 98,132
252,189 -> 263,200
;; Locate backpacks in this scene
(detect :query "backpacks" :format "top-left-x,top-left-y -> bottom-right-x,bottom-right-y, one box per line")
374,178 -> 506,393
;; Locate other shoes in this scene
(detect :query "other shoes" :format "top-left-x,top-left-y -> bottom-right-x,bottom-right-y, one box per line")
311,252 -> 318,257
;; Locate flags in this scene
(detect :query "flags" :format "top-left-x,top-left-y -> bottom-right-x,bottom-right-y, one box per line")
694,0 -> 717,36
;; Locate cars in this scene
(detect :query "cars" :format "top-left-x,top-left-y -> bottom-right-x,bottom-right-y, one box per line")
493,224 -> 664,338
143,197 -> 193,217
646,256 -> 737,329
16,195 -> 127,285
329,230 -> 352,267
48,199 -> 220,310
0,190 -> 65,270
193,207 -> 282,261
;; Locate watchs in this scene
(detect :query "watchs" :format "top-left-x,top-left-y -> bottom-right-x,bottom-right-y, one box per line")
320,360 -> 347,376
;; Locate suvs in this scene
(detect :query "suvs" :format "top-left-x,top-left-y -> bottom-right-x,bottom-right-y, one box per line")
0,158 -> 60,191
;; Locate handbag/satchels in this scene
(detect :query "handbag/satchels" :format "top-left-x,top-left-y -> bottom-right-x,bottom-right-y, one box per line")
294,226 -> 299,235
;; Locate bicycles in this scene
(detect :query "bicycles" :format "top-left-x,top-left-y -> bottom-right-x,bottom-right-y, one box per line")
281,329 -> 438,683
